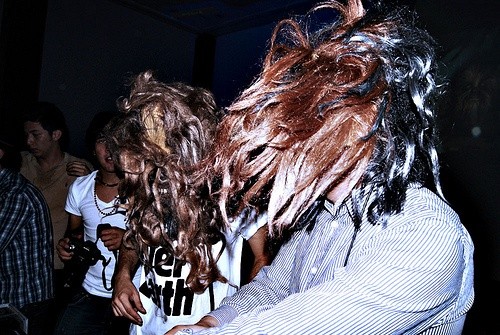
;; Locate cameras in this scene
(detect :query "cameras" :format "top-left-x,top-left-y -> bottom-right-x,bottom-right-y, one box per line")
53,241 -> 101,304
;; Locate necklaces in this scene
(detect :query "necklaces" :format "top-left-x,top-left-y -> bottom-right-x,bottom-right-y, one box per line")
95,178 -> 119,188
93,188 -> 119,215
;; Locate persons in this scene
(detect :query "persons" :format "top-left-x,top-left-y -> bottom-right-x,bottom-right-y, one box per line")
164,0 -> 475,335
0,72 -> 267,335
